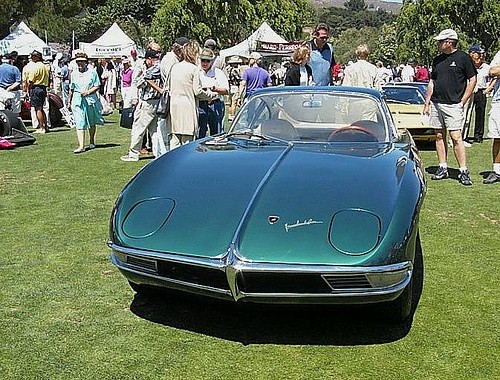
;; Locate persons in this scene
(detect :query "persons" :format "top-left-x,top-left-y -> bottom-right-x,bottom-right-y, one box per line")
0,51 -> 24,113
424,29 -> 478,185
56,58 -> 70,107
225,56 -> 242,123
238,52 -> 272,124
305,23 -> 336,123
468,45 -> 498,142
283,47 -> 313,119
92,37 -> 230,162
68,52 -> 105,153
483,49 -> 500,184
343,44 -> 382,120
22,50 -> 49,133
376,59 -> 428,82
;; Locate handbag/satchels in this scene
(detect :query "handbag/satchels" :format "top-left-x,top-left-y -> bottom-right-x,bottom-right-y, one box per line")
156,62 -> 176,119
120,105 -> 136,129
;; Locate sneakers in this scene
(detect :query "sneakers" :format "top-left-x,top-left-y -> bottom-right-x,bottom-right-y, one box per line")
456,169 -> 472,185
483,171 -> 500,184
432,166 -> 449,180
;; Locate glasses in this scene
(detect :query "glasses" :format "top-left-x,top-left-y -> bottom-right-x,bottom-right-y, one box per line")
318,35 -> 329,39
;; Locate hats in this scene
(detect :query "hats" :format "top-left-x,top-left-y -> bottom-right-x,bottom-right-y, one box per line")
175,37 -> 188,46
75,56 -> 87,60
200,49 -> 215,59
4,53 -> 15,60
433,29 -> 458,40
144,50 -> 159,58
131,50 -> 136,56
469,45 -> 485,53
408,59 -> 414,65
29,50 -> 43,59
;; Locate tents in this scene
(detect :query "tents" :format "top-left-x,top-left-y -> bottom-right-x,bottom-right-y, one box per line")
0,20 -> 54,56
72,22 -> 136,58
220,21 -> 303,63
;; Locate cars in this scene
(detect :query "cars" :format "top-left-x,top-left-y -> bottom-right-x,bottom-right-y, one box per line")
386,81 -> 429,104
381,85 -> 437,142
106,86 -> 428,323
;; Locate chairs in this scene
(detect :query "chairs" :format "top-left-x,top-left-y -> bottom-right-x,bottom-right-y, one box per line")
397,91 -> 413,102
251,119 -> 300,141
347,120 -> 386,142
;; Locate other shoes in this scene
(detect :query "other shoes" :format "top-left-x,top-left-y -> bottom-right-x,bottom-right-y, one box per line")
120,155 -> 139,161
89,143 -> 95,149
74,148 -> 85,153
33,129 -> 46,134
140,148 -> 149,155
